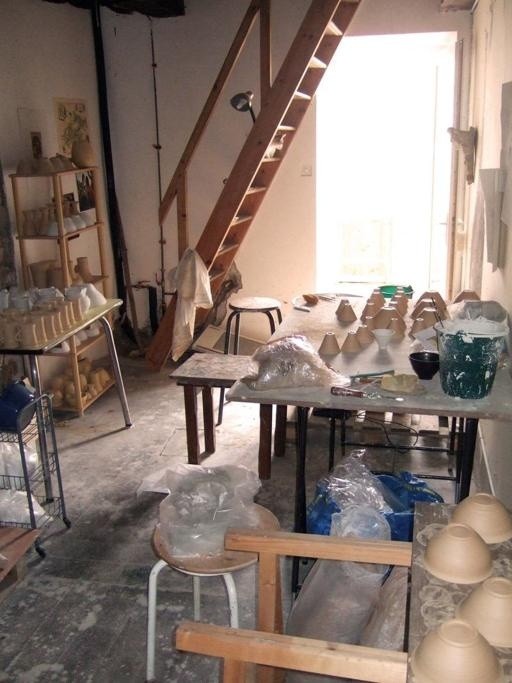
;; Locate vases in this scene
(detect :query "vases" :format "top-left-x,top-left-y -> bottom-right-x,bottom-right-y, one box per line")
71,141 -> 96,168
0,379 -> 36,433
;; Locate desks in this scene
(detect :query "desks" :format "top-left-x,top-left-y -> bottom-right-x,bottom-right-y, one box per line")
0,299 -> 133,502
225,281 -> 512,596
175,500 -> 512,683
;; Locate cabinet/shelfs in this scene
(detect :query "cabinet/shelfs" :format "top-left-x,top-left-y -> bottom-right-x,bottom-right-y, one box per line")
8,165 -> 117,416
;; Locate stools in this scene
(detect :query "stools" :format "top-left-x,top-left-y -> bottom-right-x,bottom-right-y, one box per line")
168,353 -> 287,479
219,297 -> 282,425
146,502 -> 281,682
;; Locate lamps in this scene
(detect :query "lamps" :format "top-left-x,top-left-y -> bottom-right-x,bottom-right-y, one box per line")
231,89 -> 255,122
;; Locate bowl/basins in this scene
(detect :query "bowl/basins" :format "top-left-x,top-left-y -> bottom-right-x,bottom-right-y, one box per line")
454,576 -> 511,649
454,492 -> 512,544
366,289 -> 481,337
420,522 -> 495,586
408,351 -> 441,381
411,619 -> 505,683
16,155 -> 74,176
46,210 -> 94,237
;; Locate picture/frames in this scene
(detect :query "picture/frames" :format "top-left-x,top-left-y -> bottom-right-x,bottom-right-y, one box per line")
52,96 -> 90,158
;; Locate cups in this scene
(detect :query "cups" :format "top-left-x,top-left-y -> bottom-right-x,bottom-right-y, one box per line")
317,285 -> 426,356
0,284 -> 106,349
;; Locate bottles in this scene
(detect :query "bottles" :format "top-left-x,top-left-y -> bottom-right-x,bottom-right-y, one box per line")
22,200 -> 80,236
50,367 -> 110,407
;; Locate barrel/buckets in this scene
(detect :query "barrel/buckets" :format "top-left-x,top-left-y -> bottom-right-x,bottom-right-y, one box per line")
435,319 -> 510,399
